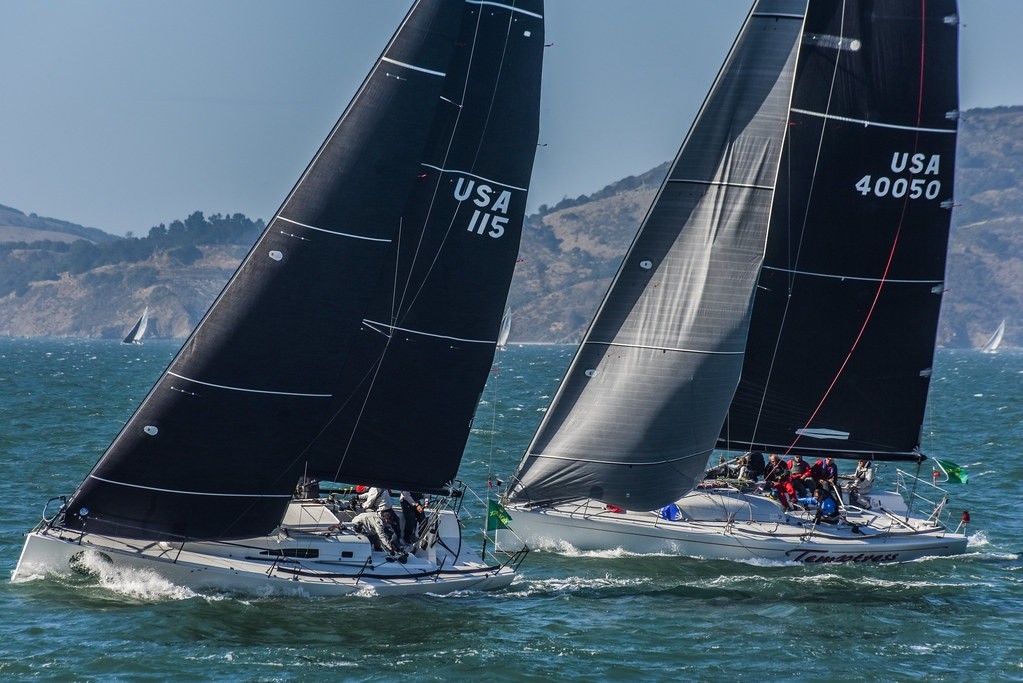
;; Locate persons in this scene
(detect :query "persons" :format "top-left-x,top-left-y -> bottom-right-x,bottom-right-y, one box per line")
351,485 -> 428,555
712,453 -> 876,525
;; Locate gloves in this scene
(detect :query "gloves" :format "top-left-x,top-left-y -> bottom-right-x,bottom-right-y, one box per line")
389,548 -> 395,556
357,495 -> 359,501
360,503 -> 363,508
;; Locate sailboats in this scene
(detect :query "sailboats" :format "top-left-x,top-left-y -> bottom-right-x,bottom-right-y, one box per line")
10,1 -> 533,602
979,319 -> 1006,354
118,303 -> 150,346
488,1 -> 974,562
494,308 -> 512,352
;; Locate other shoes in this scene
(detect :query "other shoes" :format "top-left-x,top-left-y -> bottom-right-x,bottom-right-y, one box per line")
863,506 -> 871,510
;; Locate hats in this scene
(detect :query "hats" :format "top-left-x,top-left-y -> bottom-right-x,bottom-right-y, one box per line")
383,512 -> 393,523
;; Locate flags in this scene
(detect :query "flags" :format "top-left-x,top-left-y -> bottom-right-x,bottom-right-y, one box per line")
935,456 -> 968,485
487,499 -> 514,531
